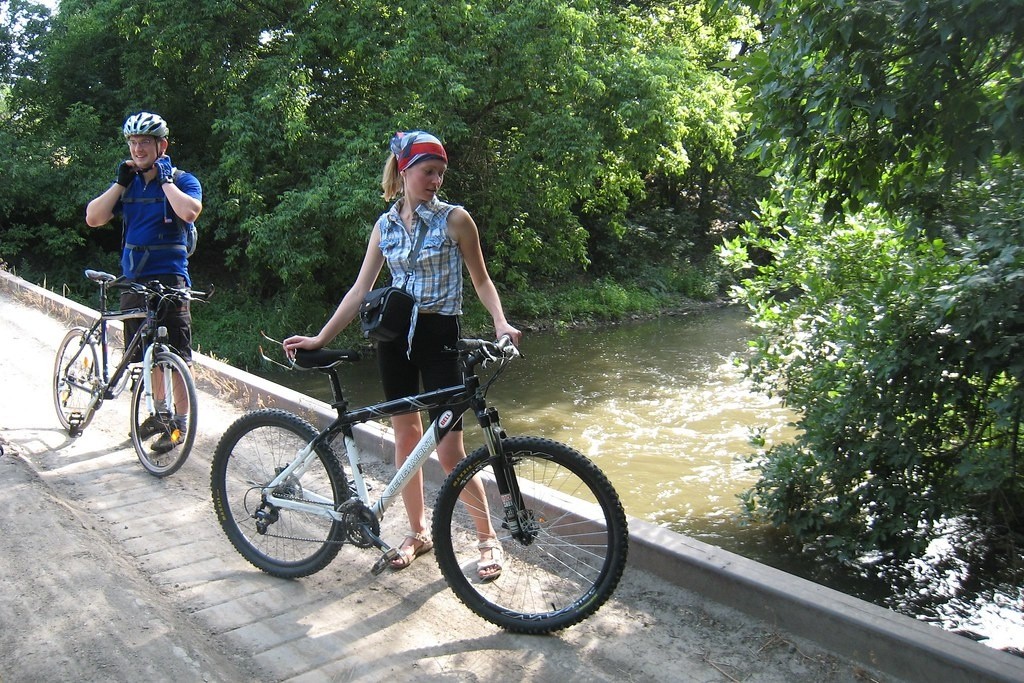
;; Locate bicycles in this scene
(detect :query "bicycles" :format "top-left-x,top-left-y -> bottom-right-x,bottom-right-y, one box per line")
208,335 -> 629,635
49,270 -> 217,480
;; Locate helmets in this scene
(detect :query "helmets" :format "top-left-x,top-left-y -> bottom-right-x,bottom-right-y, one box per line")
124,112 -> 169,138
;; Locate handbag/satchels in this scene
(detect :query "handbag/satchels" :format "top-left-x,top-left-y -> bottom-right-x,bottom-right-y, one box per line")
358,287 -> 414,342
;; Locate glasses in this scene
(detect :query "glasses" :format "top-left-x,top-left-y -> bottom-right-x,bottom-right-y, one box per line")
259,331 -> 297,370
126,139 -> 158,150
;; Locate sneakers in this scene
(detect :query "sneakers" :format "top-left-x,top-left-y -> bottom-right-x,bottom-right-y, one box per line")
151,420 -> 187,453
128,412 -> 172,439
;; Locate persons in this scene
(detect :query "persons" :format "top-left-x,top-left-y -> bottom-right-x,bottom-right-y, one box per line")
283,131 -> 522,580
86,112 -> 202,453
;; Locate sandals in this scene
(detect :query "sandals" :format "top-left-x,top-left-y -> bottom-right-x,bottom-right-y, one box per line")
477,537 -> 504,580
390,527 -> 434,569
